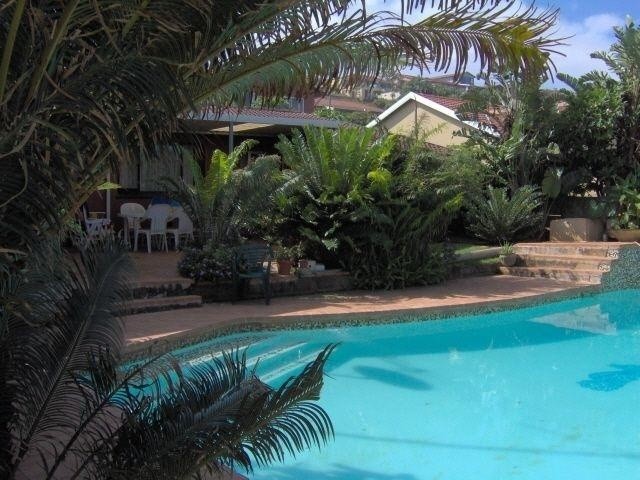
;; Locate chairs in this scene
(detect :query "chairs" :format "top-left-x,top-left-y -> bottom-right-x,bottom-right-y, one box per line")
81,202 -> 195,255
231,246 -> 272,304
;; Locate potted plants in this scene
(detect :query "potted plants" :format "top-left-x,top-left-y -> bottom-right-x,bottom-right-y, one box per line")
273,240 -> 307,276
498,242 -> 517,266
589,174 -> 640,242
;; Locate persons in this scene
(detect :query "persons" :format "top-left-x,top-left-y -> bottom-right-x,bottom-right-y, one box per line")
139,195 -> 170,231
